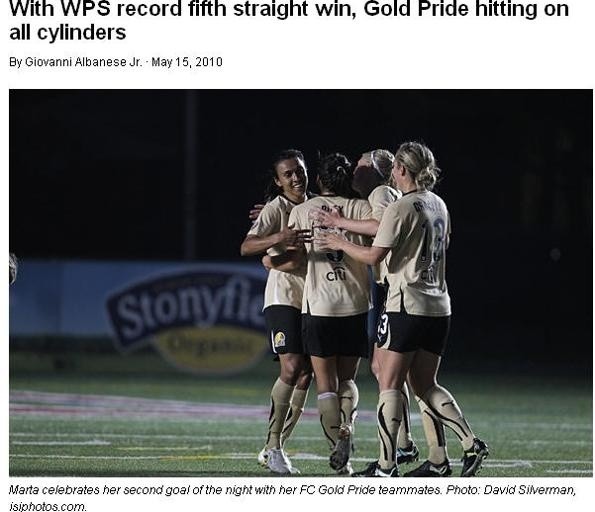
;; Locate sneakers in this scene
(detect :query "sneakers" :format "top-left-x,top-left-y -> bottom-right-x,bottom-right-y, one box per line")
403,458 -> 452,477
268,447 -> 300,475
460,438 -> 490,478
328,422 -> 353,470
351,461 -> 400,478
395,441 -> 420,465
336,462 -> 353,475
257,445 -> 269,469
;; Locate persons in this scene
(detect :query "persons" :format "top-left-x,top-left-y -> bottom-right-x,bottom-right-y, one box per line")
248,148 -> 453,477
262,151 -> 373,476
314,139 -> 490,478
239,147 -> 325,475
